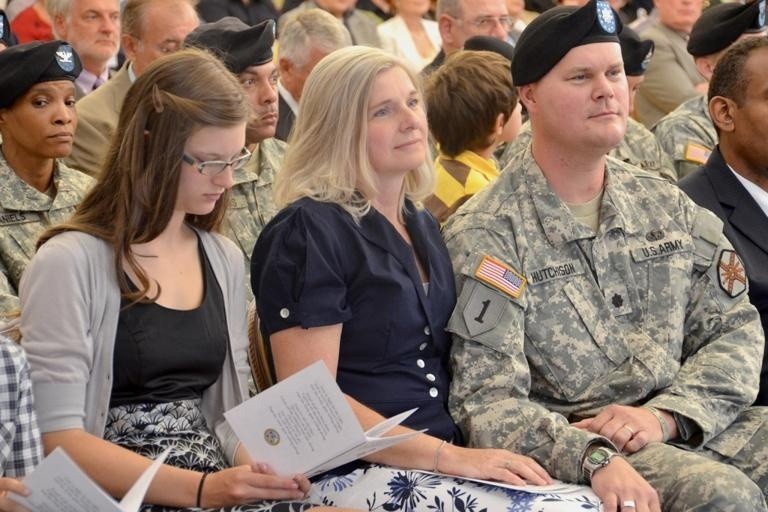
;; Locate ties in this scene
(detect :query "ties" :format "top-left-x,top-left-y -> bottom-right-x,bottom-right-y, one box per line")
92,78 -> 106,89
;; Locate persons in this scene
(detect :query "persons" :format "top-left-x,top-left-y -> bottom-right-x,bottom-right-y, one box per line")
272,9 -> 354,150
419,0 -> 511,76
0,337 -> 44,512
439,0 -> 768,512
16,2 -> 123,102
377,0 -> 441,71
619,27 -> 655,117
671,36 -> 768,409
259,1 -> 382,48
423,53 -> 522,224
11,2 -> 55,44
180,18 -> 289,264
599,0 -> 710,125
647,1 -> 768,182
0,41 -> 96,308
19,49 -> 372,512
50,2 -> 203,177
191,2 -> 280,41
249,44 -> 607,512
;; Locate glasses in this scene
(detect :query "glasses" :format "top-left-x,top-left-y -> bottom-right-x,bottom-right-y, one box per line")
177,145 -> 253,177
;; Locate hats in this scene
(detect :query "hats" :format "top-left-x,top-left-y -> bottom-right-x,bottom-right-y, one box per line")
686,0 -> 767,58
617,25 -> 655,76
0,39 -> 83,109
511,0 -> 623,87
182,15 -> 278,75
461,35 -> 530,115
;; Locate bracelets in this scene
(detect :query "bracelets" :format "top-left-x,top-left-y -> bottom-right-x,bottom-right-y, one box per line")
196,472 -> 208,510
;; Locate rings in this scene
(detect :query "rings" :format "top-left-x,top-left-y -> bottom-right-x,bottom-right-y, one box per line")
624,425 -> 634,436
621,500 -> 635,508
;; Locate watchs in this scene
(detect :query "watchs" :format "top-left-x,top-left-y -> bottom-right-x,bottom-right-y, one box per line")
581,446 -> 622,483
